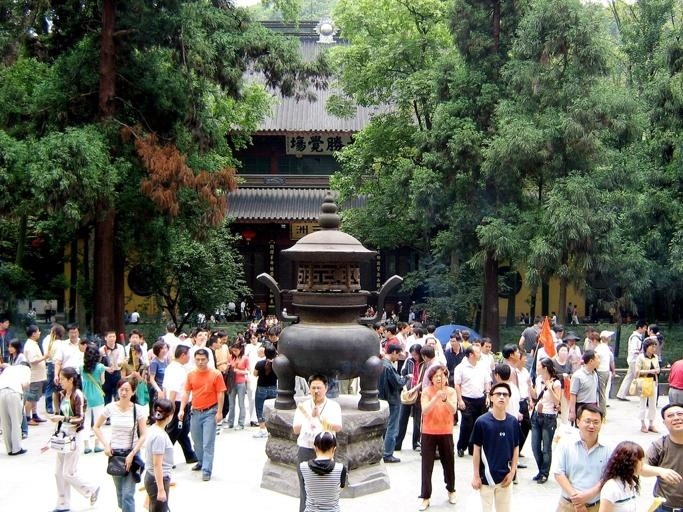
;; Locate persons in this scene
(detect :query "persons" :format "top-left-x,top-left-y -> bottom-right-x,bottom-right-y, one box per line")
36,367 -> 101,511
123,397 -> 177,510
549,402 -> 610,510
417,363 -> 459,510
366,295 -> 683,511
1,296 -> 281,511
289,373 -> 342,512
179,347 -> 226,482
467,381 -> 521,511
294,429 -> 347,511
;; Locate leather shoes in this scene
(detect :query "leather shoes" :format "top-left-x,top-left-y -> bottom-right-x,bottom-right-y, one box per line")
459,449 -> 464,457
517,464 -> 528,468
419,502 -> 430,511
8,448 -> 27,455
450,497 -> 457,504
27,420 -> 39,425
32,418 -> 47,422
384,456 -> 401,463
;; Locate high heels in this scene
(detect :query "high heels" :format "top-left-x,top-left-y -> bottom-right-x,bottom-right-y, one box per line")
251,421 -> 260,427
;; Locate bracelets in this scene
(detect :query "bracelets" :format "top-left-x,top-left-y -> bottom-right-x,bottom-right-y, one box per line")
443,397 -> 449,402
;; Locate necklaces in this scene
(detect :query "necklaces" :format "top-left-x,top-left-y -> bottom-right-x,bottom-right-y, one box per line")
318,400 -> 328,416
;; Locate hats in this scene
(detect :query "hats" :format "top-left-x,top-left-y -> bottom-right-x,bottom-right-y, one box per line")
563,331 -> 581,343
599,330 -> 615,338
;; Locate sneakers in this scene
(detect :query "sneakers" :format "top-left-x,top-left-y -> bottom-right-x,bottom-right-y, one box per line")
85,449 -> 91,453
253,428 -> 268,438
192,464 -> 202,471
94,448 -> 103,451
649,426 -> 658,433
533,473 -> 542,480
202,474 -> 210,481
234,425 -> 243,430
537,474 -> 548,484
641,426 -> 648,433
223,424 -> 229,429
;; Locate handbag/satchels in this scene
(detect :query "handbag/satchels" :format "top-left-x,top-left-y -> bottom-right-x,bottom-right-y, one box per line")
564,378 -> 570,400
400,390 -> 418,405
629,377 -> 656,397
107,456 -> 129,476
559,421 -> 580,441
135,382 -> 151,406
47,431 -> 76,453
223,365 -> 236,393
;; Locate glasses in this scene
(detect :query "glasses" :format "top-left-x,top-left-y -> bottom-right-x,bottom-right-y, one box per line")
494,393 -> 510,397
580,420 -> 600,426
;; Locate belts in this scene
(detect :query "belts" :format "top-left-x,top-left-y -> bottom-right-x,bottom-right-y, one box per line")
200,403 -> 218,412
662,504 -> 683,512
563,497 -> 600,507
669,386 -> 683,390
